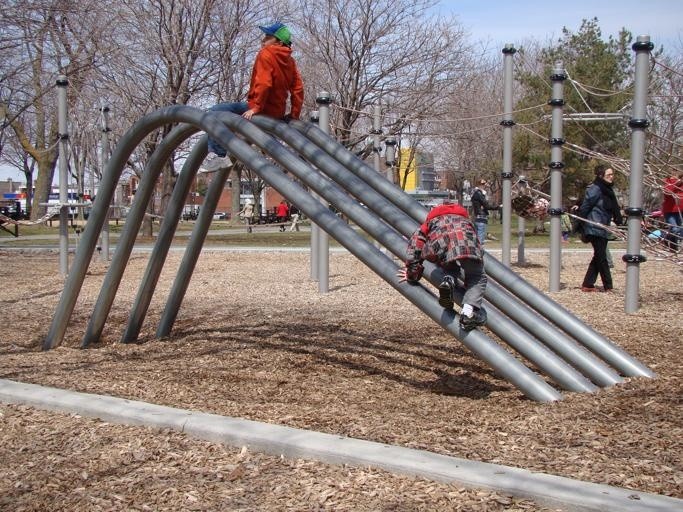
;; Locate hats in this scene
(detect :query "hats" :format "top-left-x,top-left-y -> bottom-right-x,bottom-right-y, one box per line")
258,23 -> 291,46
477,179 -> 486,184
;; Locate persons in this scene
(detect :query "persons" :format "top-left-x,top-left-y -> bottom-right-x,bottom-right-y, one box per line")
288,204 -> 301,231
471,179 -> 492,246
198,23 -> 304,172
570,161 -> 625,292
560,209 -> 573,244
276,200 -> 289,232
657,169 -> 682,253
395,201 -> 487,331
234,198 -> 254,233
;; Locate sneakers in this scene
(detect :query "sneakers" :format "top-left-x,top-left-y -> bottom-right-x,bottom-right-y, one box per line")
460,306 -> 487,330
439,277 -> 455,310
581,286 -> 599,291
200,153 -> 232,172
606,289 -> 615,292
